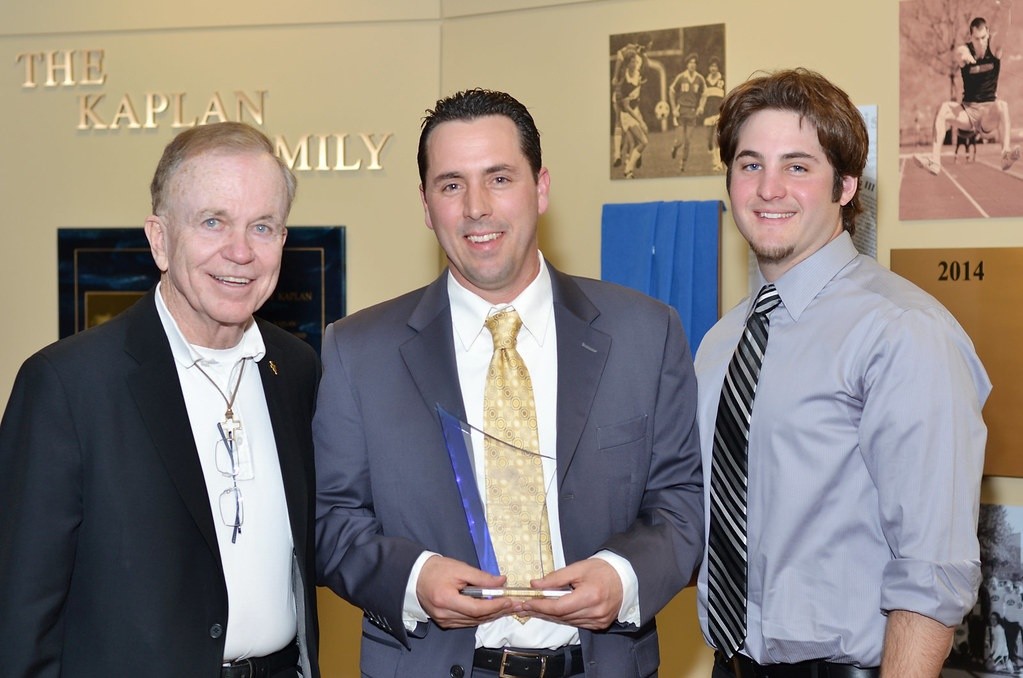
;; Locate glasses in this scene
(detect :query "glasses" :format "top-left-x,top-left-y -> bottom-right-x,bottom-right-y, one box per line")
215,422 -> 244,544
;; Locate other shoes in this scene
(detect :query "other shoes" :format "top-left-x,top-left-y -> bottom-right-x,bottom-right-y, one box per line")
1001,145 -> 1020,169
1006,660 -> 1014,675
680,158 -> 687,171
623,170 -> 634,180
913,153 -> 941,176
708,141 -> 713,152
613,156 -> 622,167
671,144 -> 681,159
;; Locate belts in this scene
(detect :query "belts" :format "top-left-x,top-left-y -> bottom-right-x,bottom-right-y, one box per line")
473,646 -> 584,678
714,650 -> 880,678
220,645 -> 299,678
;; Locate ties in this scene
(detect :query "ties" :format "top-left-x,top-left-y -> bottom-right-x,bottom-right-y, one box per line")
708,285 -> 783,662
484,310 -> 555,626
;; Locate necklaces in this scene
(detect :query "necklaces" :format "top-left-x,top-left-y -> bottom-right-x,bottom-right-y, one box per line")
193,357 -> 247,455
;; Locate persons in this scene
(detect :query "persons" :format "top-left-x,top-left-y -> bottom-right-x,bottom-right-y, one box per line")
912,0 -> 1023,178
611,31 -> 726,180
690,64 -> 995,678
0,120 -> 323,678
308,87 -> 708,678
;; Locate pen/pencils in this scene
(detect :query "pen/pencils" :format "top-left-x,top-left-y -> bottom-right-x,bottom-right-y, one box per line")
460,586 -> 572,597
